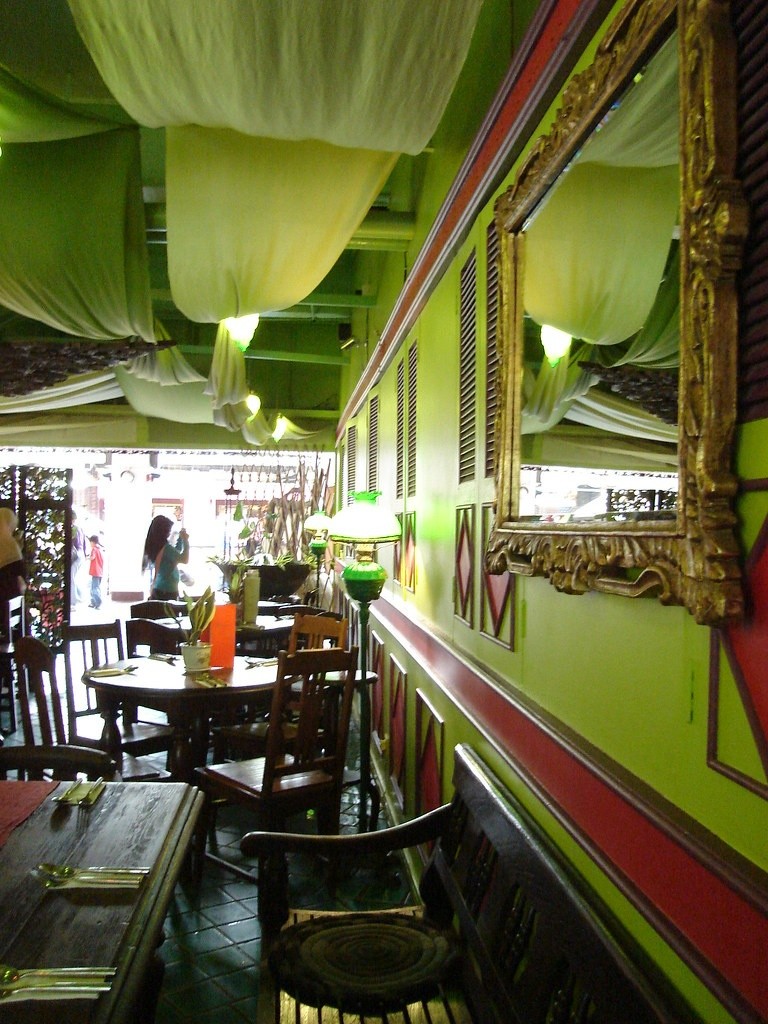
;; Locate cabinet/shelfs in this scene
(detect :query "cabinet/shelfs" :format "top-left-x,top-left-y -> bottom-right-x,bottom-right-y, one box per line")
307,669 -> 379,832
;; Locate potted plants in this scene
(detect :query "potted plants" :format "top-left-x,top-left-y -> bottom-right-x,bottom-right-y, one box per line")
223,561 -> 244,627
162,586 -> 216,671
205,553 -> 325,601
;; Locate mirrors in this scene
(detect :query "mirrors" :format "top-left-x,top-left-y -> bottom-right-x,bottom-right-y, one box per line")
483,0 -> 752,630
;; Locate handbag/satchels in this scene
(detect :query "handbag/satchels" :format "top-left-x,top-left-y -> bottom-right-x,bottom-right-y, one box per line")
70,544 -> 78,562
150,581 -> 154,595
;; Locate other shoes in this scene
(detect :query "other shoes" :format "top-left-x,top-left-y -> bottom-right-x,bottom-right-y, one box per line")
70,605 -> 76,612
88,604 -> 100,610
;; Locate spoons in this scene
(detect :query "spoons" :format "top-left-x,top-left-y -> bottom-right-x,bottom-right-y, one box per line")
51,778 -> 83,802
36,862 -> 151,878
0,964 -> 117,985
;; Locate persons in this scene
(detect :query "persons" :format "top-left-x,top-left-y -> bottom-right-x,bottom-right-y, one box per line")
87,536 -> 104,609
70,510 -> 90,605
141,515 -> 190,601
0,507 -> 23,598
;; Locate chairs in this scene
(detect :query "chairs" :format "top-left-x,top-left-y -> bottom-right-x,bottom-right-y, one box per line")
0,595 -> 359,897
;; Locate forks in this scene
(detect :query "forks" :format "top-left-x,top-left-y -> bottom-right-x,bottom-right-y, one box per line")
76,777 -> 104,805
30,869 -> 140,886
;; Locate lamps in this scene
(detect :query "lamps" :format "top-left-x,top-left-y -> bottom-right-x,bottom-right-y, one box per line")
329,490 -> 402,835
302,510 -> 332,609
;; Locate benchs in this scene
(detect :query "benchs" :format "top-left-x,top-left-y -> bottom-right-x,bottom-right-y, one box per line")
240,743 -> 705,1024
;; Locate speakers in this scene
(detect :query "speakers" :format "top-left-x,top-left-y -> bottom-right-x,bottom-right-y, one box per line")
338,323 -> 352,342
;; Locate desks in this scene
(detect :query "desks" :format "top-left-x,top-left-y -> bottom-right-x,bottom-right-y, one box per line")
80,653 -> 304,785
170,597 -> 291,616
0,781 -> 208,1024
150,614 -> 297,659
